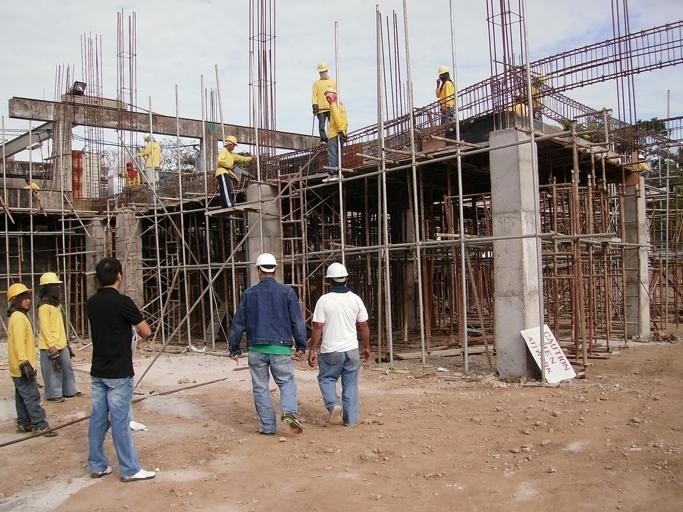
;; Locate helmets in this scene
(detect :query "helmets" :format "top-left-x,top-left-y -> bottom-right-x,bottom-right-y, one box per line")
255,253 -> 278,273
126,162 -> 133,167
325,262 -> 349,279
436,64 -> 450,75
39,272 -> 63,286
142,132 -> 149,144
225,134 -> 239,147
315,62 -> 330,72
324,86 -> 337,96
7,283 -> 32,305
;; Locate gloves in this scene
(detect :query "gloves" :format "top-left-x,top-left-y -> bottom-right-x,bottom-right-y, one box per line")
47,350 -> 63,372
19,360 -> 35,383
67,344 -> 76,359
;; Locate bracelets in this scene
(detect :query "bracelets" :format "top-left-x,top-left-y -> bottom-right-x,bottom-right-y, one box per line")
309,351 -> 318,355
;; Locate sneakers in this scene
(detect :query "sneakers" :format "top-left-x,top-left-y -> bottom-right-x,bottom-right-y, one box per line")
120,467 -> 157,483
280,412 -> 304,435
256,430 -> 277,437
322,172 -> 345,183
43,396 -> 66,405
89,465 -> 116,479
326,404 -> 344,426
32,426 -> 59,439
344,423 -> 360,429
16,422 -> 34,434
62,391 -> 85,398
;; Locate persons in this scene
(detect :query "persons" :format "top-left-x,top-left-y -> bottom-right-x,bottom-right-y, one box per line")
309,262 -> 370,427
226,252 -> 306,434
137,135 -> 161,202
5,283 -> 52,431
24,177 -> 39,190
118,162 -> 141,186
321,88 -> 347,182
85,257 -> 155,481
434,66 -> 456,124
311,62 -> 336,145
39,272 -> 83,400
507,76 -> 549,116
216,135 -> 253,207
